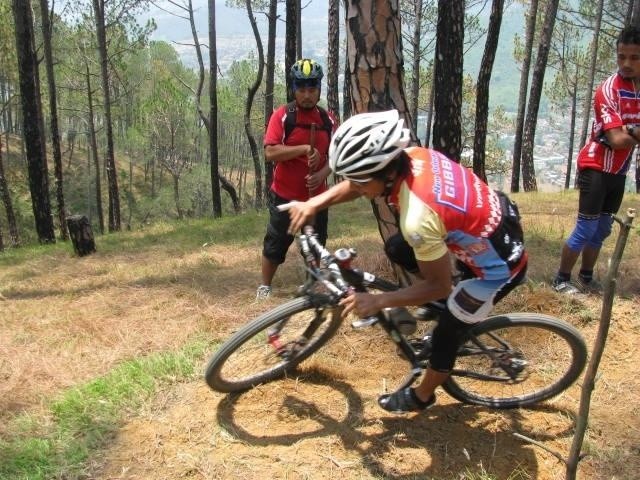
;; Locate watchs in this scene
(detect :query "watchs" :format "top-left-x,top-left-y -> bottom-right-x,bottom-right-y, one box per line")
626,123 -> 633,135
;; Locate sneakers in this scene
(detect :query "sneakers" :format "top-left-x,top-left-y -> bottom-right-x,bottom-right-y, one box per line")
415,308 -> 440,322
577,276 -> 600,290
552,278 -> 579,296
255,284 -> 272,299
378,386 -> 435,412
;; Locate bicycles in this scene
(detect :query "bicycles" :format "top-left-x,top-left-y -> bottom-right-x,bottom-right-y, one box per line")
207,200 -> 586,410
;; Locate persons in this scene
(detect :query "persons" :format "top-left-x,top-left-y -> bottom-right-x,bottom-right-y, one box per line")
254,58 -> 339,300
550,25 -> 639,298
287,108 -> 527,413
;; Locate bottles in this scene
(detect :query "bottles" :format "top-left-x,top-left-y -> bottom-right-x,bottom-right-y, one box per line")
383,304 -> 417,335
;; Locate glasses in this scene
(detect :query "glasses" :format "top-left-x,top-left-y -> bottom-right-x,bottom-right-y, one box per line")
343,176 -> 374,187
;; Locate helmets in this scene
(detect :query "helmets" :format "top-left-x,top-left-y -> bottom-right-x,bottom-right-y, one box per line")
289,58 -> 324,82
328,109 -> 410,175
